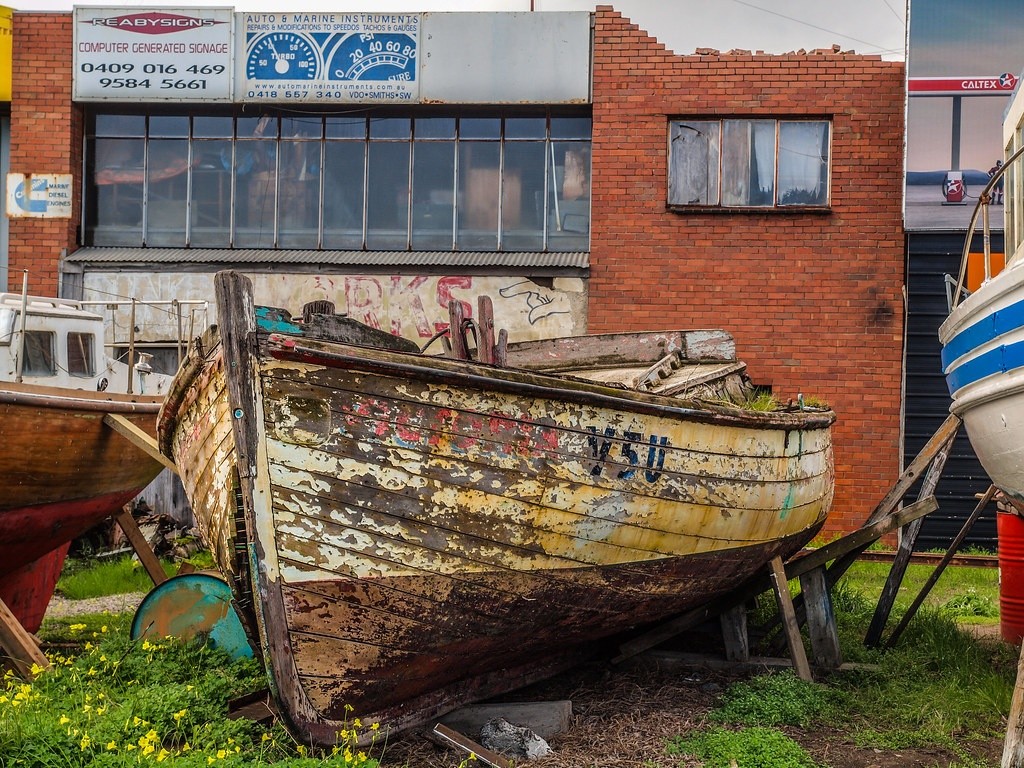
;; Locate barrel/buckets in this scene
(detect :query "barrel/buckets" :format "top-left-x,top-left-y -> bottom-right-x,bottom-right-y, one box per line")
997,513 -> 1024,647
947,172 -> 962,202
129,571 -> 254,659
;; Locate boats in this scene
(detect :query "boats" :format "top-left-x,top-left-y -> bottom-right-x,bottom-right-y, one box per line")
0,265 -> 207,679
152,267 -> 839,756
938,73 -> 1024,517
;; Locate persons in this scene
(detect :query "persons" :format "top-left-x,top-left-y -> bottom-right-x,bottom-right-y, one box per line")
986,160 -> 1004,205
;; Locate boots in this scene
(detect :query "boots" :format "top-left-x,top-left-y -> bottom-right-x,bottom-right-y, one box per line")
997,194 -> 1003,205
990,192 -> 996,205
